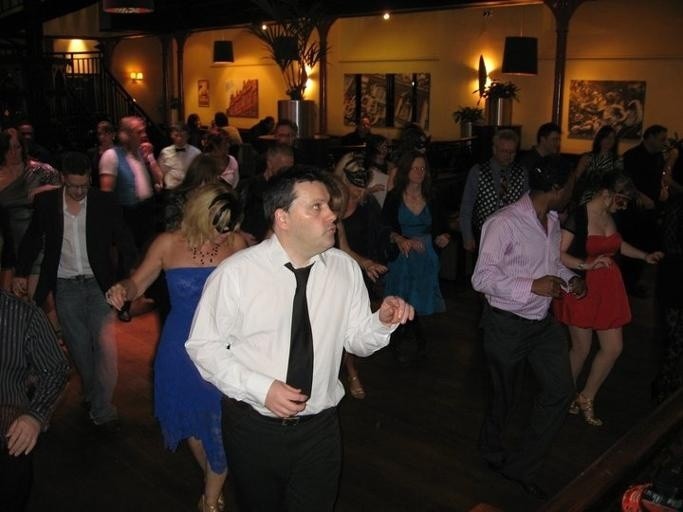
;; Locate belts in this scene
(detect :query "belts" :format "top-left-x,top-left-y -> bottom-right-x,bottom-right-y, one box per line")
277,415 -> 316,425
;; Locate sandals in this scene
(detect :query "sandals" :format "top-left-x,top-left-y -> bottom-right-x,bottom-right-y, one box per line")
348,370 -> 366,399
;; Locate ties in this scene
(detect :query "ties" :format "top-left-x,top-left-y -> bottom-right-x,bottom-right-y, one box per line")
286,262 -> 313,404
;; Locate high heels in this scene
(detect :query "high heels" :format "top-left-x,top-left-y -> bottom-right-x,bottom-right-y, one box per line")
569,391 -> 603,426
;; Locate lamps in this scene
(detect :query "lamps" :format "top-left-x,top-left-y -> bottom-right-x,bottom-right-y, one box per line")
211,28 -> 234,66
500,4 -> 542,77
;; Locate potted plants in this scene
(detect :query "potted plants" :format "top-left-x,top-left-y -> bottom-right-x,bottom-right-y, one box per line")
158,95 -> 180,127
243,2 -> 336,138
481,80 -> 516,126
452,108 -> 480,147
